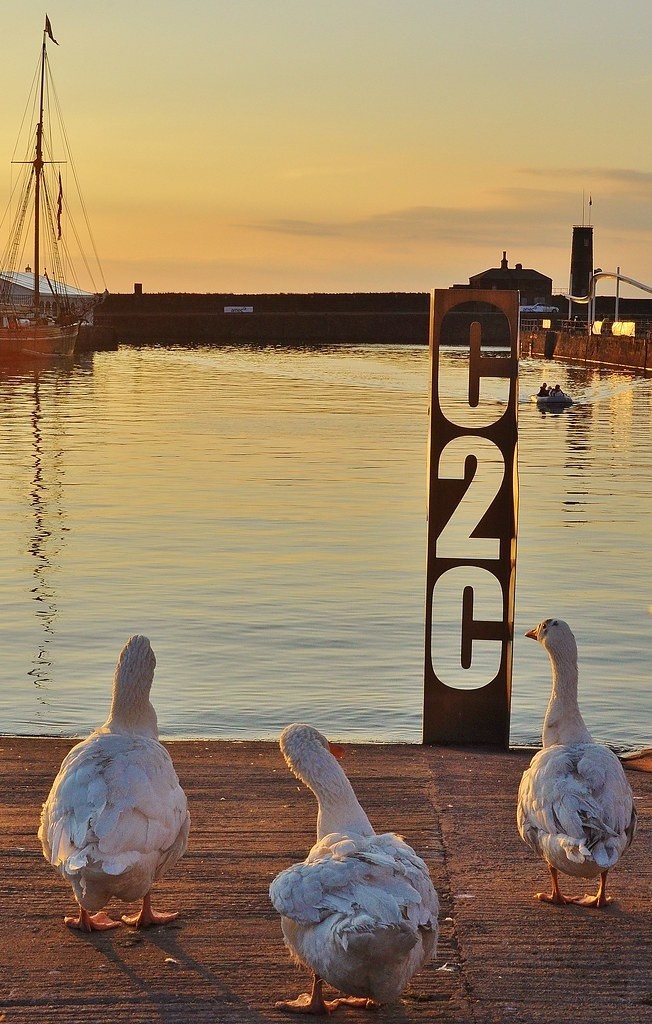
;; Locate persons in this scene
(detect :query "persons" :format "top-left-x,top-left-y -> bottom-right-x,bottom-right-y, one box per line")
537,383 -> 564,397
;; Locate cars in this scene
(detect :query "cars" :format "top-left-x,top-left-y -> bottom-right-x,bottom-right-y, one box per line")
532,303 -> 560,313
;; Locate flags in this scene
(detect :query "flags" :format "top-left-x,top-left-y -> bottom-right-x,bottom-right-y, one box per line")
45,14 -> 60,47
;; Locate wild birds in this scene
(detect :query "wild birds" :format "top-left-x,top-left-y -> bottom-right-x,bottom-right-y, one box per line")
37,635 -> 191,933
267,724 -> 441,1014
517,618 -> 637,907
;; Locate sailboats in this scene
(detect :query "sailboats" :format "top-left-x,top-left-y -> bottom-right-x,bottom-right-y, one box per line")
1,12 -> 109,359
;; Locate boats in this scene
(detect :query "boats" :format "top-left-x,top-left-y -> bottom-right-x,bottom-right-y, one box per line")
535,394 -> 572,403
